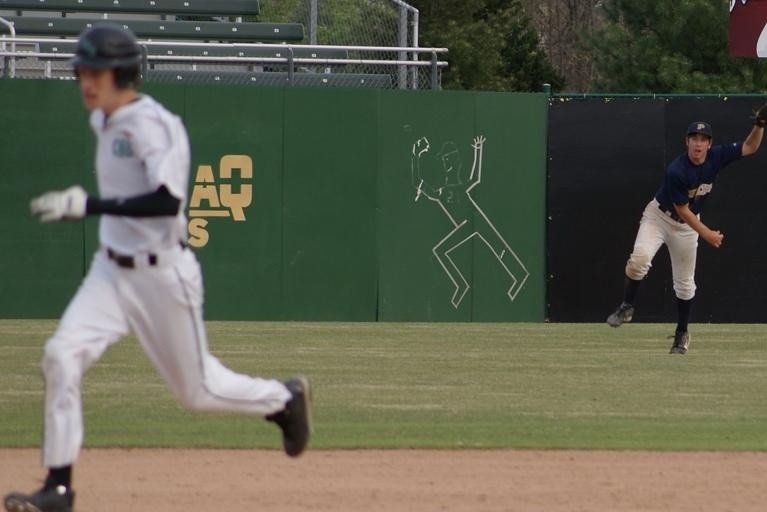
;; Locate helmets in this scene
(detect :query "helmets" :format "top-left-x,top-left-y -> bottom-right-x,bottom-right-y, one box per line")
71,27 -> 144,88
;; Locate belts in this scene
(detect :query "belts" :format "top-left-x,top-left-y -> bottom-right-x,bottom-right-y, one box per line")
658,204 -> 684,224
107,239 -> 184,267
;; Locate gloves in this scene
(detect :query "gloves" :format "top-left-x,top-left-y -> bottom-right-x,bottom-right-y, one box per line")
29,184 -> 87,223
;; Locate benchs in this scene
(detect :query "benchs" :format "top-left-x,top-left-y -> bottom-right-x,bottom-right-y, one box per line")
0,0 -> 393,86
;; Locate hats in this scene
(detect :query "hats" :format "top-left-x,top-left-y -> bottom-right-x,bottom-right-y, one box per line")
686,121 -> 712,138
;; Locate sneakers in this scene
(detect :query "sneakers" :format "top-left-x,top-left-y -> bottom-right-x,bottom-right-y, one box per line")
266,376 -> 311,457
606,301 -> 634,327
4,479 -> 75,511
669,326 -> 690,353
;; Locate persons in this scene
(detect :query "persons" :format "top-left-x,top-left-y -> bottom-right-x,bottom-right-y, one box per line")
607,100 -> 766,355
4,21 -> 313,511
